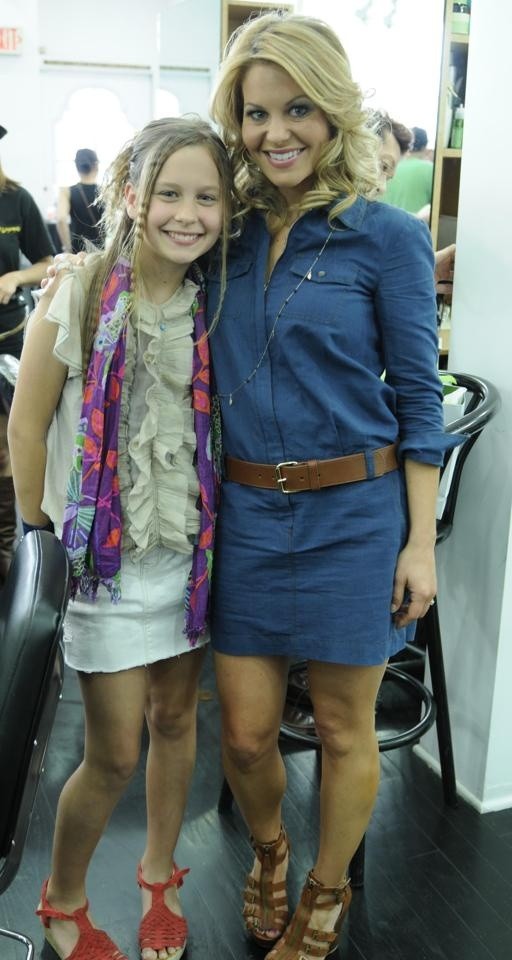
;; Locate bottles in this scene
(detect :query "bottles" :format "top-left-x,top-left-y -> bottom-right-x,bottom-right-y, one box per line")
449,103 -> 464,150
452,0 -> 470,37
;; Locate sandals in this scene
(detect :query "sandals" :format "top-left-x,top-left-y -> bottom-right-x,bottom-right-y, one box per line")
264,868 -> 352,960
241,824 -> 288,941
35,877 -> 127,960
137,863 -> 189,960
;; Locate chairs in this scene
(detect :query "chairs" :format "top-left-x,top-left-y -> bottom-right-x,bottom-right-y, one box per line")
217,369 -> 501,894
0,528 -> 75,960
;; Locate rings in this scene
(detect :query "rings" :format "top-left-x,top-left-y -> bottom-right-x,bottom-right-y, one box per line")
428,598 -> 436,608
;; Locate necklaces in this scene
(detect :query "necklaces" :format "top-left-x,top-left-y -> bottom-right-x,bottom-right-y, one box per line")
212,225 -> 333,404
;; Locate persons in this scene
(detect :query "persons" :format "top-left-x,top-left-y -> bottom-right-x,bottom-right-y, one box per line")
357,107 -> 457,304
7,116 -> 236,960
36,15 -> 459,960
380,127 -> 434,219
0,129 -> 58,409
50,149 -> 111,255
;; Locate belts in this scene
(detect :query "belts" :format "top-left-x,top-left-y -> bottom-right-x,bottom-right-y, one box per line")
224,443 -> 400,493
